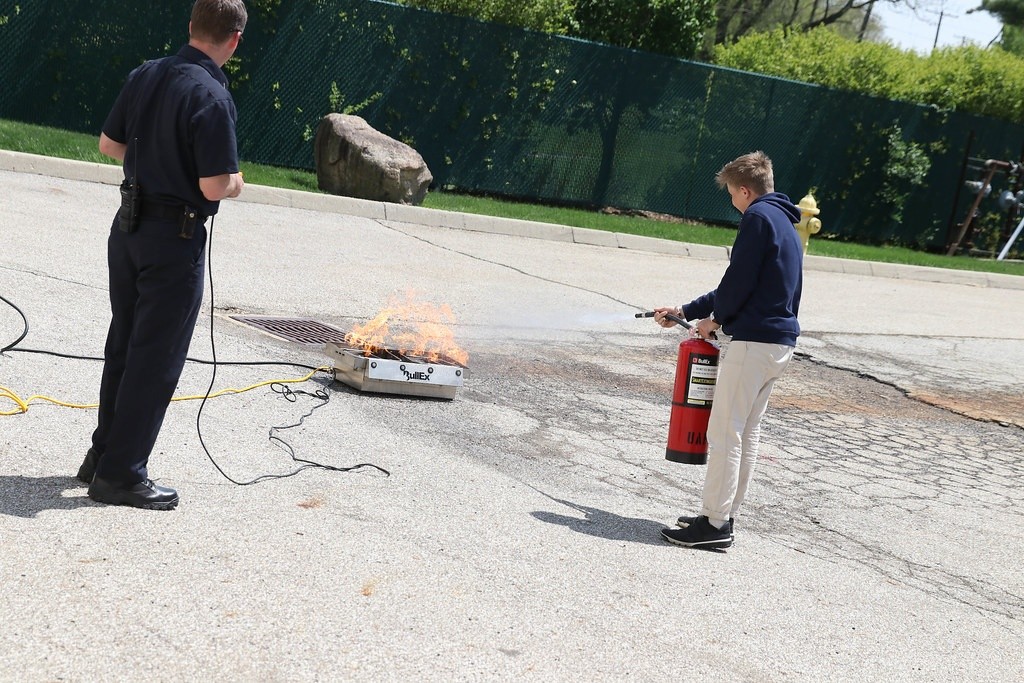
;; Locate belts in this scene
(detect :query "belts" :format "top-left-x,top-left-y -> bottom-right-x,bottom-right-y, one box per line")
138,199 -> 208,223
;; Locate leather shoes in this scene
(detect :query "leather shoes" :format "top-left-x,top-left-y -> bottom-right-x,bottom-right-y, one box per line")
87,471 -> 180,511
77,448 -> 100,483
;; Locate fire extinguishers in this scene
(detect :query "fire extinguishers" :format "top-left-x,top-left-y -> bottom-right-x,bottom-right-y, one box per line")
635,311 -> 721,466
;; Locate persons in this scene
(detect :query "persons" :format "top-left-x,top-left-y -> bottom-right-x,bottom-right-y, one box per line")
73,1 -> 249,509
651,149 -> 805,549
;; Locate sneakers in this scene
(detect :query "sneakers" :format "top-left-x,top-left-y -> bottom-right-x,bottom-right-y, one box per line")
661,515 -> 732,548
677,516 -> 734,542
